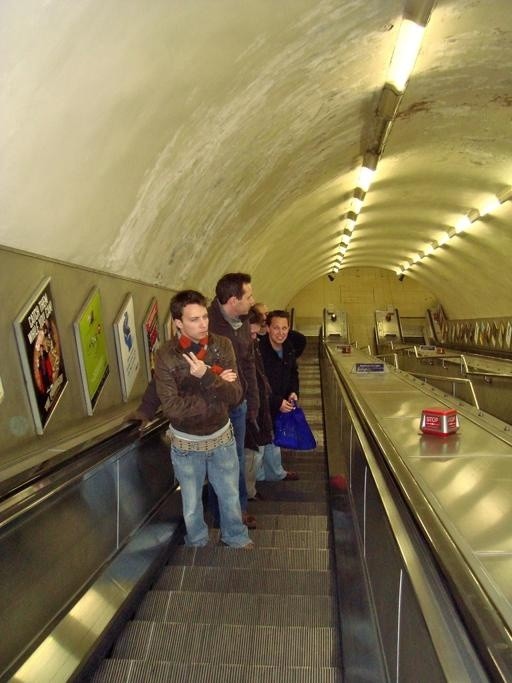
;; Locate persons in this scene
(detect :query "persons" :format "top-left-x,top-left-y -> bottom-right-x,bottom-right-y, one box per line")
238,307 -> 276,501
38,343 -> 55,392
259,307 -> 299,484
251,301 -> 306,359
122,270 -> 261,529
155,291 -> 260,547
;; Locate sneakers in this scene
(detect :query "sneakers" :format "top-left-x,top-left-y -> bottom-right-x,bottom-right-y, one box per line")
283,470 -> 299,480
241,512 -> 257,529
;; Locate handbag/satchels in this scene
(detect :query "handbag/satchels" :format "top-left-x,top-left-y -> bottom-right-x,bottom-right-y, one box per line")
273,401 -> 316,449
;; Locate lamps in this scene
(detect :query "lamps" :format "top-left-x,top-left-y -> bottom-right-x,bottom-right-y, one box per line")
328,1 -> 511,285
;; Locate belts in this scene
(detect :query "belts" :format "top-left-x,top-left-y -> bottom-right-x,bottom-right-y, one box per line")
170,423 -> 234,451
